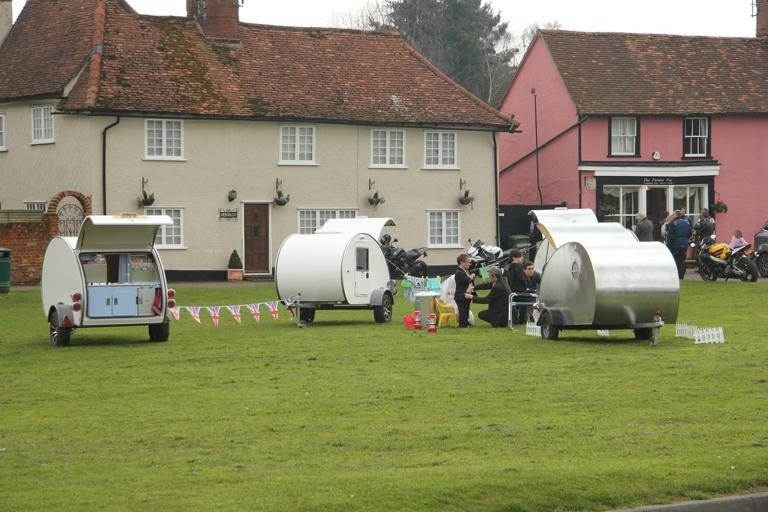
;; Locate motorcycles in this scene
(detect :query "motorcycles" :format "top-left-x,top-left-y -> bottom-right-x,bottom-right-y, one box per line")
686,232 -> 762,283
463,236 -> 533,278
377,233 -> 431,280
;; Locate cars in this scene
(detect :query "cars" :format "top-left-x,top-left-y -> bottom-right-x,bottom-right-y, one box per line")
753,219 -> 768,278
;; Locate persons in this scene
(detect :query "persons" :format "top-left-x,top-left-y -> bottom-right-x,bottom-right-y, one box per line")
454,248 -> 541,329
630,206 -> 717,280
728,229 -> 748,251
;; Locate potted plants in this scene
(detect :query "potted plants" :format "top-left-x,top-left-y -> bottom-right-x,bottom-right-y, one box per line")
458,179 -> 475,207
135,176 -> 156,209
226,249 -> 244,280
597,204 -> 617,217
711,198 -> 729,214
273,178 -> 290,206
367,177 -> 385,208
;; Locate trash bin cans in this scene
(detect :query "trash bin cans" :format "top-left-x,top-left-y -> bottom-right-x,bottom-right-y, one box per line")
0,248 -> 12,294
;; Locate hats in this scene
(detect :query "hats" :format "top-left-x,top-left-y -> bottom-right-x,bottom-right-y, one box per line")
486,266 -> 502,275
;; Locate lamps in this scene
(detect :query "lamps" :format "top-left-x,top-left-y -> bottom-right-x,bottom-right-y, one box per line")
227,190 -> 237,202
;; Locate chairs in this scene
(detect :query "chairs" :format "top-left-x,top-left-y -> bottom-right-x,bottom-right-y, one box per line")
433,298 -> 458,329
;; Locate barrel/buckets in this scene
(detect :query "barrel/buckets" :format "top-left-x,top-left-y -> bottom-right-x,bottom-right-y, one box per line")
413,291 -> 441,328
